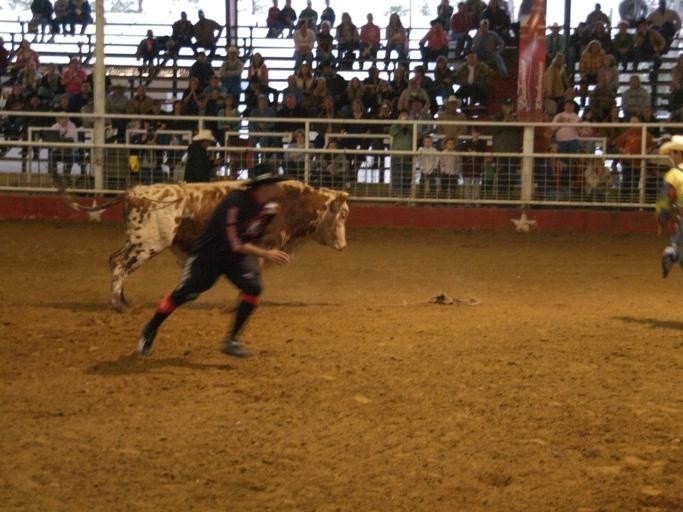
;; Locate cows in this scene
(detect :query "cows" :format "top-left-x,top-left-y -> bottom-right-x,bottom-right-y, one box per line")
55,174 -> 350,313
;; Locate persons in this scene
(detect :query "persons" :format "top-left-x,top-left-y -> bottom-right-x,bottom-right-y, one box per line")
45,113 -> 79,187
135,10 -> 223,66
419,0 -> 511,76
657,135 -> 683,279
1,38 -> 94,156
137,160 -> 291,358
32,1 -> 93,42
294,12 -> 409,75
534,0 -> 683,206
266,1 -> 336,40
98,47 -> 524,206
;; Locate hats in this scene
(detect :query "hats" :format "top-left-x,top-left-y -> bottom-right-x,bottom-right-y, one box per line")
226,46 -> 239,55
240,162 -> 285,187
193,128 -> 216,142
409,97 -> 427,108
548,23 -> 563,30
443,95 -> 461,108
657,134 -> 682,155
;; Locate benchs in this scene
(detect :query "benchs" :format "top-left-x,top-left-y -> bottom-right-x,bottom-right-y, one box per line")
0,16 -> 681,190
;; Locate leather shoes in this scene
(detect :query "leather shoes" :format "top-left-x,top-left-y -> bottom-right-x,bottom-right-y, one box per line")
220,339 -> 247,357
136,325 -> 154,354
